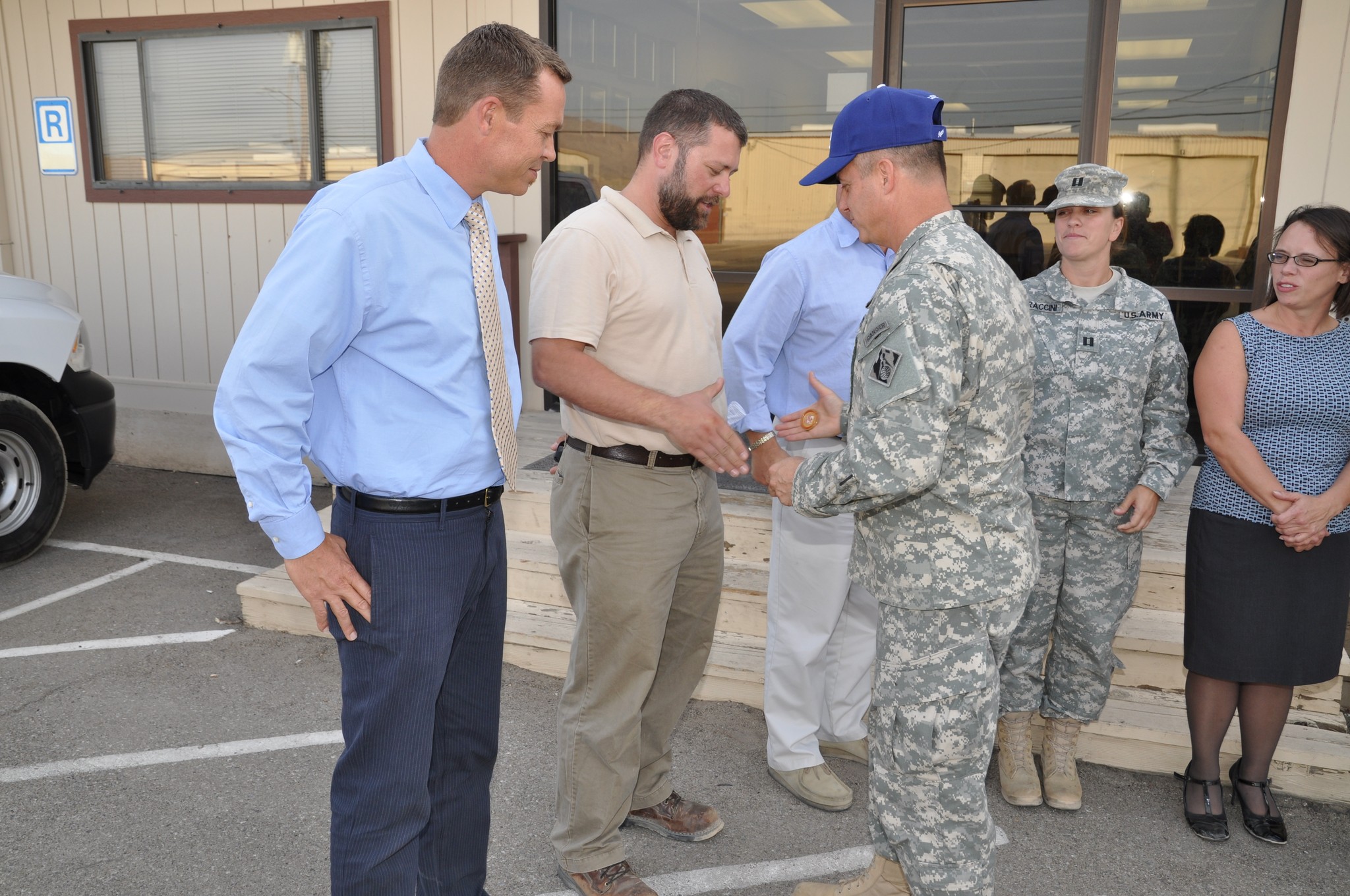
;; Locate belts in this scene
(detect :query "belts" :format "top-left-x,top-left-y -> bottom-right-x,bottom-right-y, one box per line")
566,433 -> 707,470
340,484 -> 504,515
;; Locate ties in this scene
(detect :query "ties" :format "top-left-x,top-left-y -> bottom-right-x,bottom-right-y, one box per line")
464,202 -> 518,494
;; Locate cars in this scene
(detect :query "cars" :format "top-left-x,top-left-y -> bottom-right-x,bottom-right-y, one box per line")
0,273 -> 118,570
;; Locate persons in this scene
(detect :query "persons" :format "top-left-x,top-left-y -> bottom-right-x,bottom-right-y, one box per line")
767,83 -> 1043,896
525,88 -> 750,896
214,25 -> 571,896
1177,206 -> 1350,845
719,185 -> 900,812
998,164 -> 1199,810
960,175 -> 1259,467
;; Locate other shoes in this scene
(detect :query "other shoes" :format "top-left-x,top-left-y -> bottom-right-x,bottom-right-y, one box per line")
818,733 -> 869,765
768,759 -> 853,811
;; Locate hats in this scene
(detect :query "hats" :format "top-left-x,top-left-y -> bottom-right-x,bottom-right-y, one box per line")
799,84 -> 947,186
1044,163 -> 1128,213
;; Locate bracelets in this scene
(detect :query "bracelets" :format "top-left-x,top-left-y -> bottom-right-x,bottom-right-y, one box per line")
749,430 -> 776,452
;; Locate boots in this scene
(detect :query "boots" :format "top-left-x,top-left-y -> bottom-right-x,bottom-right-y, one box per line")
997,708 -> 1042,806
792,853 -> 911,896
1042,719 -> 1084,810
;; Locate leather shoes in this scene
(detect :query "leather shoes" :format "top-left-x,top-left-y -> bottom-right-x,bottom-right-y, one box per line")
558,858 -> 657,896
621,787 -> 725,843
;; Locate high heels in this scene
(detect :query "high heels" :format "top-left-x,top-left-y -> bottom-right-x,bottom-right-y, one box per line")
1174,759 -> 1229,842
1228,758 -> 1287,845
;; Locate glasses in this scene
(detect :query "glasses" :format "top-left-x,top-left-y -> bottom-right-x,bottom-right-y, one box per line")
1268,253 -> 1347,268
